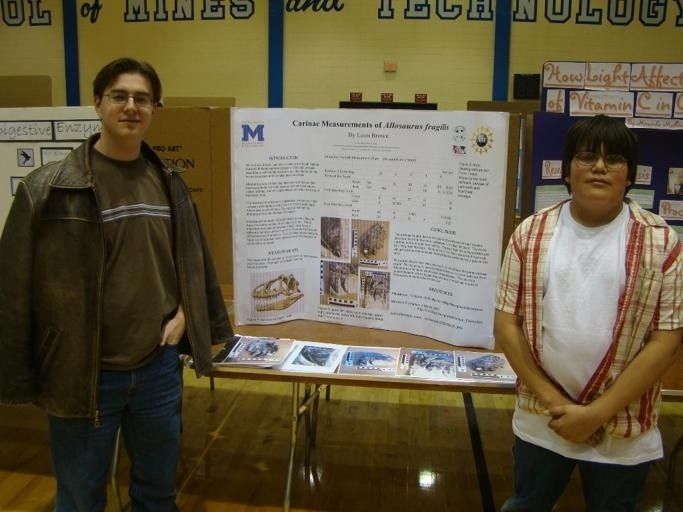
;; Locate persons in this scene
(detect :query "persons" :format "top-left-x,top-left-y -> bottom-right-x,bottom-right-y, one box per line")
0,56 -> 237,511
491,114 -> 682,512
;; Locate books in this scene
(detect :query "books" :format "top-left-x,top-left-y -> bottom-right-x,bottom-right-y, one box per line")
335,343 -> 402,379
393,347 -> 456,384
208,334 -> 295,366
281,340 -> 349,376
446,349 -> 518,385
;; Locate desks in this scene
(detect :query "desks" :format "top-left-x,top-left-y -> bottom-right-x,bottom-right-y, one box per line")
196,328 -> 517,512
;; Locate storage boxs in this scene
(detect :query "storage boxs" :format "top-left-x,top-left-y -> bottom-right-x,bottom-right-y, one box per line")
116,104 -> 231,290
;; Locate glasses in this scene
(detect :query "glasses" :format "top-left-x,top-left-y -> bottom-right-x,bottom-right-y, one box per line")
102,91 -> 155,108
573,151 -> 631,170
